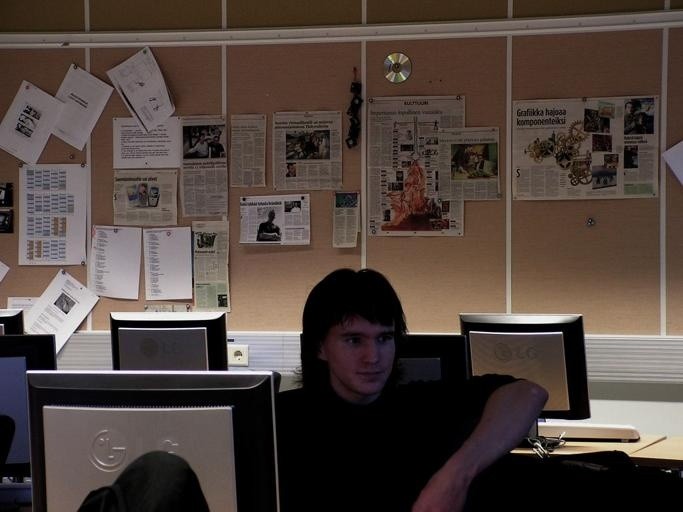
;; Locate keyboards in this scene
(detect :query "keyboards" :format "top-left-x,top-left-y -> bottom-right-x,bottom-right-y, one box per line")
536,423 -> 641,442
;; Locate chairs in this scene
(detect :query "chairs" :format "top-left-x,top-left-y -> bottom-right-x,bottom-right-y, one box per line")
457,445 -> 646,512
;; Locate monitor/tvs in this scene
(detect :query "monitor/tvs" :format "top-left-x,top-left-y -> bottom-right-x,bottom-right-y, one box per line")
27,371 -> 283,512
299,331 -> 470,385
109,310 -> 229,369
0,335 -> 56,476
0,308 -> 24,336
459,311 -> 591,447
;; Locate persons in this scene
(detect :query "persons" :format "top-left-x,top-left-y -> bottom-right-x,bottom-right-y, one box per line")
291,202 -> 301,212
276,268 -> 548,511
257,210 -> 281,241
625,101 -> 637,134
632,112 -> 654,134
286,164 -> 296,177
468,145 -> 497,178
186,126 -> 225,158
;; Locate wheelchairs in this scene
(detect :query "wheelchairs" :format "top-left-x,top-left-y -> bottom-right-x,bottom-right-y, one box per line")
567,151 -> 593,185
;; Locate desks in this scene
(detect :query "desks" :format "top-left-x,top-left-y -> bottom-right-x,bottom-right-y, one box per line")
490,422 -> 682,472
